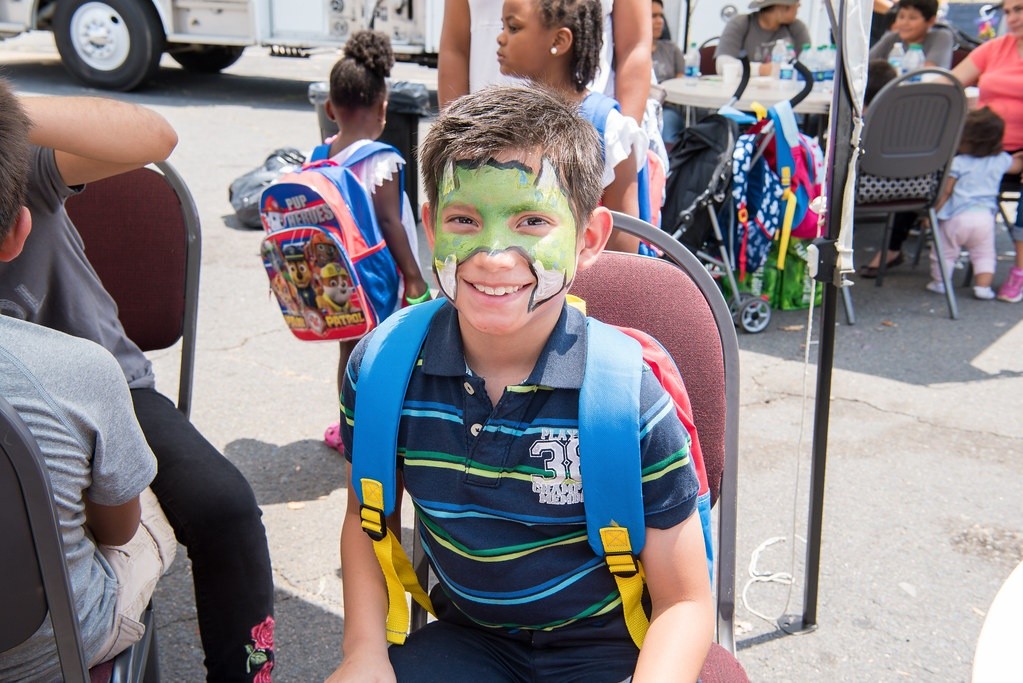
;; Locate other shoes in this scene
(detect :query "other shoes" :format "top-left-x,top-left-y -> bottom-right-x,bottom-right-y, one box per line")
324,425 -> 349,456
860,249 -> 903,279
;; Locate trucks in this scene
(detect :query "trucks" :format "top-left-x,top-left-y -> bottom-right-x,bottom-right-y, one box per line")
0,0 -> 449,94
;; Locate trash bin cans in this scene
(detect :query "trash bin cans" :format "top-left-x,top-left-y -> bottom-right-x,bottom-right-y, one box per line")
308,80 -> 429,227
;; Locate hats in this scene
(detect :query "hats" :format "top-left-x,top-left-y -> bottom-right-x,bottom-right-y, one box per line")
749,0 -> 798,9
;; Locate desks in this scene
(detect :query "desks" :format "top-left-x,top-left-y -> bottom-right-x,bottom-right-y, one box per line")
661,77 -> 978,116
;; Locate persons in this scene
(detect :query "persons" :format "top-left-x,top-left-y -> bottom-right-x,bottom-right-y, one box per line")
713,0 -> 811,77
0,94 -> 277,683
323,85 -> 713,683
925,107 -> 1023,299
651,0 -> 686,152
0,78 -> 176,683
496,0 -> 650,254
306,30 -> 435,453
860,0 -> 1023,302
439,0 -> 653,259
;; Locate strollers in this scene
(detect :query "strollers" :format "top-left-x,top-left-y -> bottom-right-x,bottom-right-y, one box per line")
654,47 -> 815,335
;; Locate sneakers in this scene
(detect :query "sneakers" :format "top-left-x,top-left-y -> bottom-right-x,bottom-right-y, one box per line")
998,270 -> 1023,304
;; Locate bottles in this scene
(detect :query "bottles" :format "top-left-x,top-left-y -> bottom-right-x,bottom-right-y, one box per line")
902,44 -> 924,85
887,43 -> 905,74
771,38 -> 837,94
684,42 -> 701,85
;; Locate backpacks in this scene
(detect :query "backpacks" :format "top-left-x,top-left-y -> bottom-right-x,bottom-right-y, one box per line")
259,141 -> 410,342
751,100 -> 829,240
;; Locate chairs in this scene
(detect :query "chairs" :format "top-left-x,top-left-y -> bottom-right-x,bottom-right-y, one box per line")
409,209 -> 751,683
839,65 -> 1021,326
932,24 -> 982,69
697,37 -> 719,75
0,160 -> 202,683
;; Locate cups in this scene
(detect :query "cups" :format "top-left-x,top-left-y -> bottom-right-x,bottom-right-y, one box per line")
723,65 -> 738,87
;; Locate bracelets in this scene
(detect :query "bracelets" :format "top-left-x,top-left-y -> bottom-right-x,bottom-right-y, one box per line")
406,282 -> 430,304
